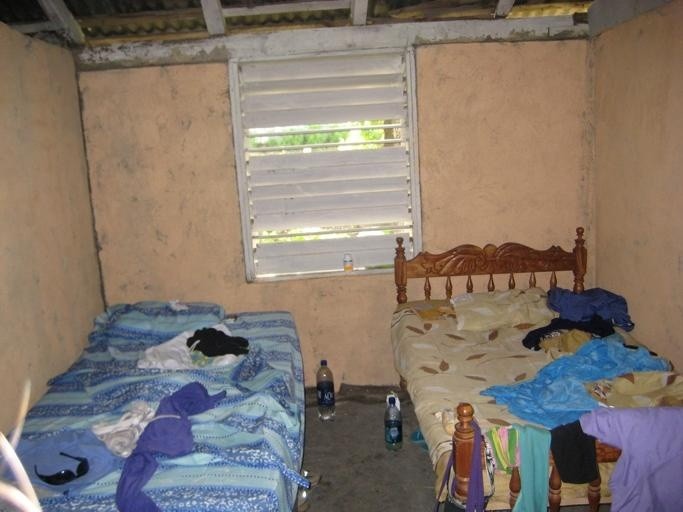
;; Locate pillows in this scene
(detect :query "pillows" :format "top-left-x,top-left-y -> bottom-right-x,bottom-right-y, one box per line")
453,288 -> 558,329
99,305 -> 227,337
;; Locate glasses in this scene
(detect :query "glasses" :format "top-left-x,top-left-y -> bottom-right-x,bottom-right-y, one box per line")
35,452 -> 89,485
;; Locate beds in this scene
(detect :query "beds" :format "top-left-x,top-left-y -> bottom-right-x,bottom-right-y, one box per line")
1,303 -> 308,512
391,227 -> 682,512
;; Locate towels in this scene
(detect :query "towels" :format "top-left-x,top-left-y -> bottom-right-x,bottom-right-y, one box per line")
513,422 -> 552,512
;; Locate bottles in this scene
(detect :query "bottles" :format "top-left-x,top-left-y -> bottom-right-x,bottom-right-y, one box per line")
342,254 -> 353,277
384,397 -> 403,451
314,359 -> 336,422
